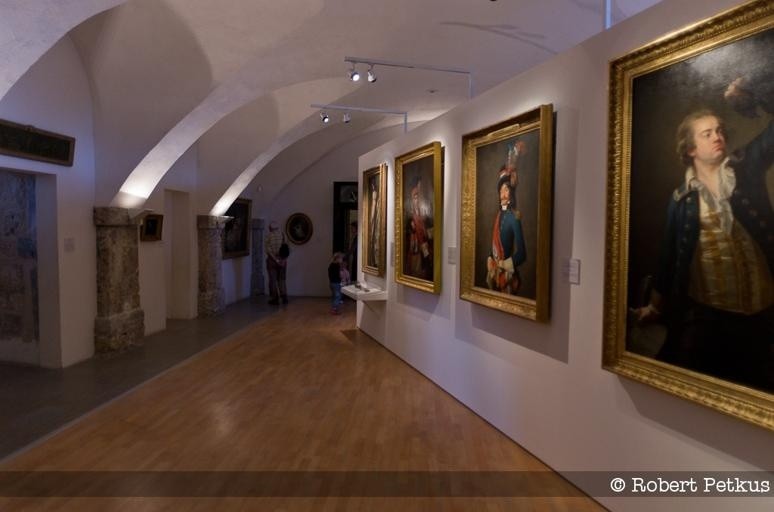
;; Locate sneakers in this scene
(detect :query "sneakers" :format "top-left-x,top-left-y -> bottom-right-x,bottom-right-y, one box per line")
328,307 -> 342,316
267,297 -> 289,306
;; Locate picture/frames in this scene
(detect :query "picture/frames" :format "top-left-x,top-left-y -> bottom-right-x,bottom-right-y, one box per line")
600,0 -> 772,429
220,198 -> 252,259
141,214 -> 163,241
457,104 -> 552,322
362,163 -> 385,277
393,141 -> 445,295
285,212 -> 314,245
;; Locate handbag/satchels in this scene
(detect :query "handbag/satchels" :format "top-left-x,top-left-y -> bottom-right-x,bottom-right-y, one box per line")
278,230 -> 290,260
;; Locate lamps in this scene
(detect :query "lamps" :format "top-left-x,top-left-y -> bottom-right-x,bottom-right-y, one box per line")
313,104 -> 408,134
344,57 -> 475,100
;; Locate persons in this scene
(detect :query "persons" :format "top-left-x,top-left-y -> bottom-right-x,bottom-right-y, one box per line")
404,182 -> 433,279
327,252 -> 345,315
485,182 -> 527,295
342,221 -> 357,283
339,261 -> 351,304
265,221 -> 289,305
629,75 -> 772,393
